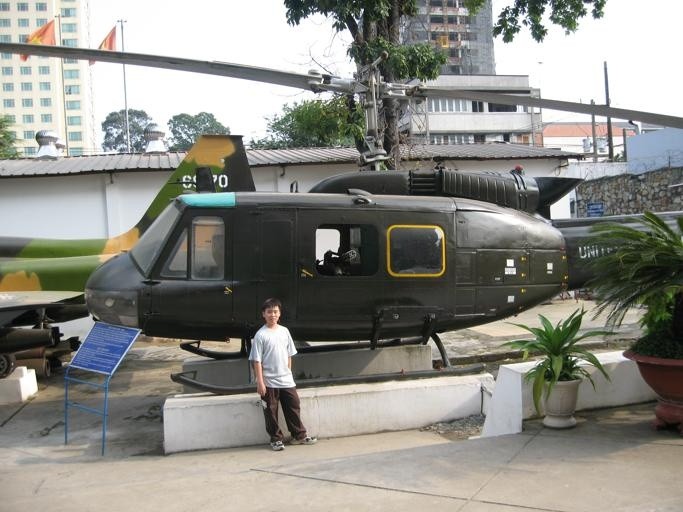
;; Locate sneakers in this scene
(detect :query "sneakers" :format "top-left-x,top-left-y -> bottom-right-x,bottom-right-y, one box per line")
289,435 -> 317,446
270,440 -> 285,451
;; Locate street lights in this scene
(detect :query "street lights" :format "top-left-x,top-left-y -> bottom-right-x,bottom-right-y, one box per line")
527,61 -> 544,78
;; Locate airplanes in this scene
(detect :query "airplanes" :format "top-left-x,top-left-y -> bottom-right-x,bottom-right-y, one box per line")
0,130 -> 259,390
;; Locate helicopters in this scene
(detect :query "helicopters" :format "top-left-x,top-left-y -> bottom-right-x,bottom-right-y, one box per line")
0,38 -> 682,393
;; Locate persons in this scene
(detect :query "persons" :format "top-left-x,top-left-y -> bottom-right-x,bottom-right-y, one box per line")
248,296 -> 316,451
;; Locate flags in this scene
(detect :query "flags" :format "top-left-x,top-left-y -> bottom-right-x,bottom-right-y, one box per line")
20,18 -> 57,61
88,24 -> 117,67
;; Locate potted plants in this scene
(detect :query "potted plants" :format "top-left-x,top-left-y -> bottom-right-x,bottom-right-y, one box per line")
496,310 -> 613,427
574,203 -> 682,436
